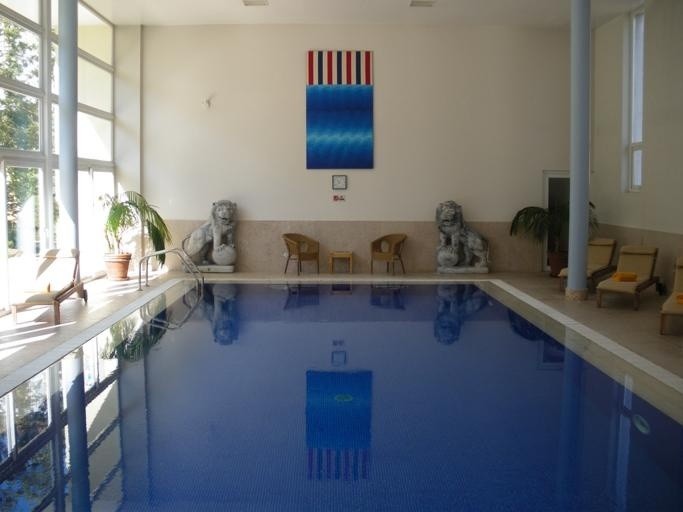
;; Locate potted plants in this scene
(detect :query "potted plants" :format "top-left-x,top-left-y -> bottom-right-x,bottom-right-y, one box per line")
96,189 -> 171,283
505,197 -> 601,278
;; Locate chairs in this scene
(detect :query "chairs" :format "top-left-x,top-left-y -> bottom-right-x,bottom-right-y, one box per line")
593,244 -> 665,311
281,282 -> 325,313
556,236 -> 616,297
368,285 -> 409,314
278,229 -> 323,278
658,254 -> 683,336
9,246 -> 88,327
367,231 -> 408,277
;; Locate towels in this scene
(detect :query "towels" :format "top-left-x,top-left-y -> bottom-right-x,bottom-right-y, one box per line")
673,292 -> 682,307
611,270 -> 636,283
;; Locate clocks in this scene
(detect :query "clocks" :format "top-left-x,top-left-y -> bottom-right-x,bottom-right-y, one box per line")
330,349 -> 349,366
331,175 -> 347,190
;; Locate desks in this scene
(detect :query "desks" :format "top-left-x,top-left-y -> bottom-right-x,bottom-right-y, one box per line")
326,249 -> 355,277
326,282 -> 355,296
